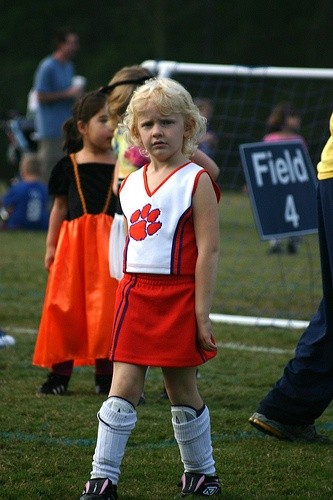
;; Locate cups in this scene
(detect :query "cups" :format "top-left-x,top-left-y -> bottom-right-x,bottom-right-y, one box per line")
72,76 -> 87,91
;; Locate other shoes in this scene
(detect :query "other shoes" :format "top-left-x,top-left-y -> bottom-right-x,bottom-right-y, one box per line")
95,379 -> 112,394
79,478 -> 117,500
248,411 -> 331,446
180,472 -> 221,498
39,377 -> 69,394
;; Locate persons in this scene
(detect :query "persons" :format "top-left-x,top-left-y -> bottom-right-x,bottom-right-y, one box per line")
0,94 -> 217,209
29,86 -> 122,396
32,21 -> 83,171
245,111 -> 333,444
78,76 -> 221,500
260,100 -> 304,256
102,64 -> 225,281
